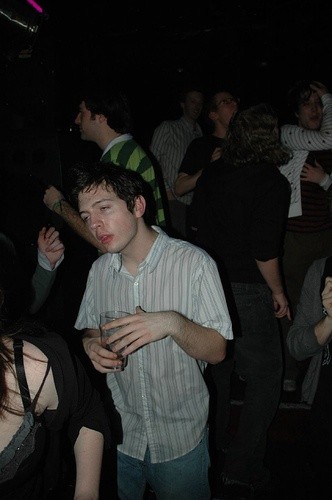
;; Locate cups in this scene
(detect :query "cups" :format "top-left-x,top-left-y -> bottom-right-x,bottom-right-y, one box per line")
100,311 -> 132,368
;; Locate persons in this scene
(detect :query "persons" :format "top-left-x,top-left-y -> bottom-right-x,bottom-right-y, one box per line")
0,64 -> 332,500
72,157 -> 235,500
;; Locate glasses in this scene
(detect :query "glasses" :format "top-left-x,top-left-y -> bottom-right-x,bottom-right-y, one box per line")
213,97 -> 240,111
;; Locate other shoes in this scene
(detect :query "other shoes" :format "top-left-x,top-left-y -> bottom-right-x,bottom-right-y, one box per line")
280,380 -> 298,392
222,466 -> 275,488
